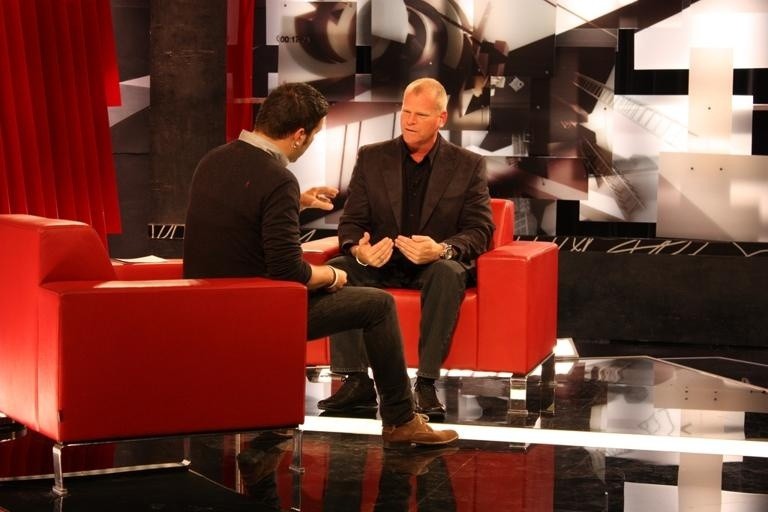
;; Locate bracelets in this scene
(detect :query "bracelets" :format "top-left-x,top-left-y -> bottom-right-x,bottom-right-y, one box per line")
355,253 -> 368,267
327,263 -> 338,289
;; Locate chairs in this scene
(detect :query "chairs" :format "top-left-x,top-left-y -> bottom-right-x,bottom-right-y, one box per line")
300,198 -> 559,410
1,213 -> 308,494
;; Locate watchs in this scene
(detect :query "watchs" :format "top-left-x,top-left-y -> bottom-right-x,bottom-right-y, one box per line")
440,241 -> 454,260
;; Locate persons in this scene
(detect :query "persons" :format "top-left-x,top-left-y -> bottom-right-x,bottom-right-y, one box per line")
181,81 -> 459,451
316,76 -> 496,420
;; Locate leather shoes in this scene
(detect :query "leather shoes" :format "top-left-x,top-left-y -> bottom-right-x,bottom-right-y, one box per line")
317,376 -> 379,412
381,415 -> 457,449
414,383 -> 447,418
